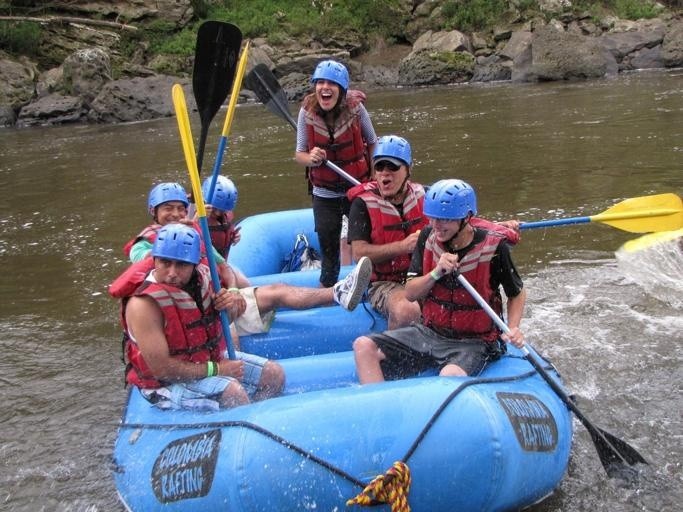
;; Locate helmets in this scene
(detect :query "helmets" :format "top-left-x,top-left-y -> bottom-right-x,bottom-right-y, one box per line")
150,224 -> 202,264
422,177 -> 478,220
311,60 -> 351,90
371,133 -> 413,167
146,181 -> 190,211
200,174 -> 239,212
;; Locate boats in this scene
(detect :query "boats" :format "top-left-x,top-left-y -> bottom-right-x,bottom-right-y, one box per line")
111,208 -> 571,512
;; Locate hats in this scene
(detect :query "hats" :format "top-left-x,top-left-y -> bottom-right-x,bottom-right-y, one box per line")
373,158 -> 401,168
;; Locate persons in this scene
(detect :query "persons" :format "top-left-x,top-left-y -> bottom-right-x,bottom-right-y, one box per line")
348,134 -> 442,331
187,176 -> 242,255
352,179 -> 525,383
109,226 -> 285,411
126,180 -> 372,351
293,57 -> 375,286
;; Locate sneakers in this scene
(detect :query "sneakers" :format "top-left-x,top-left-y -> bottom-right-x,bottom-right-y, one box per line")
331,256 -> 374,310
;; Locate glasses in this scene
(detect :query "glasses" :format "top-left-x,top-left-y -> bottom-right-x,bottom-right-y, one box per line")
429,217 -> 450,224
374,163 -> 398,172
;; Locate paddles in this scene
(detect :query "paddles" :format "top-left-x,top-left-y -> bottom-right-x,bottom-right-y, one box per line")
192,20 -> 242,203
243,63 -> 298,133
451,269 -> 648,481
503,192 -> 683,234
171,83 -> 238,362
205,40 -> 249,205
